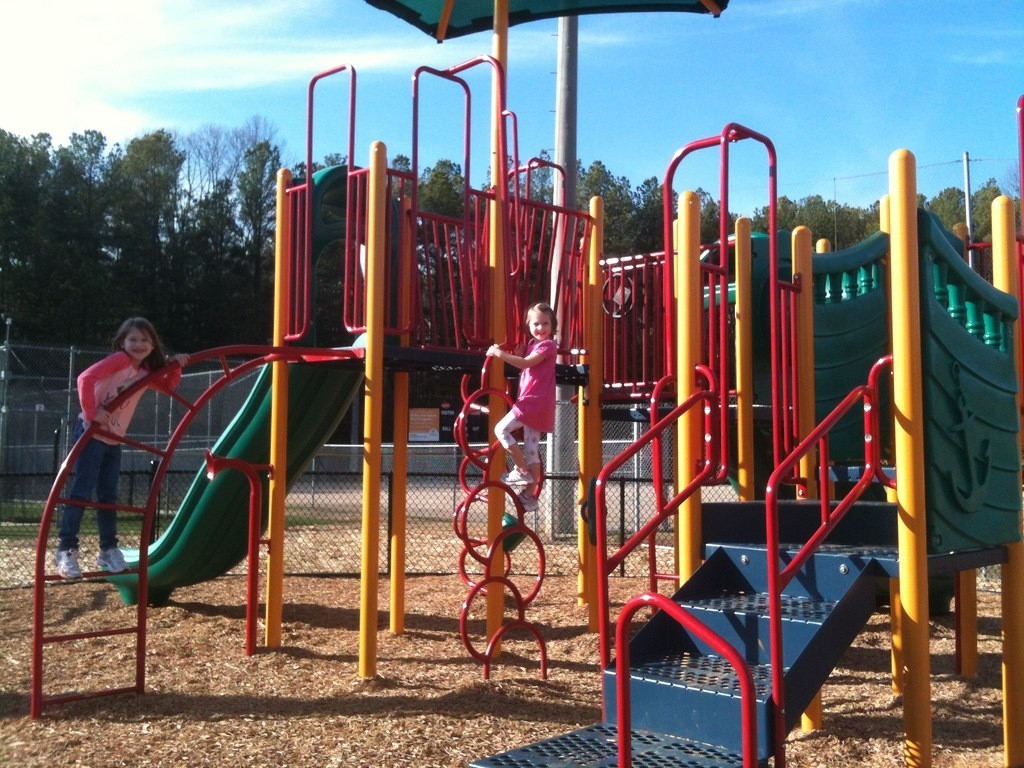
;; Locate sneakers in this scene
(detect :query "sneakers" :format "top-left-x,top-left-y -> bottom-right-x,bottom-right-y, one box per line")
96,547 -> 131,574
506,489 -> 539,511
500,466 -> 535,486
52,549 -> 83,579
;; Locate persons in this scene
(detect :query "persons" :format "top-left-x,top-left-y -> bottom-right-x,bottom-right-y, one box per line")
486,303 -> 558,511
54,318 -> 190,578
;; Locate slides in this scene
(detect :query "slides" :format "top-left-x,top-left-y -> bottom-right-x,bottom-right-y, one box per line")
101,359 -> 366,605
